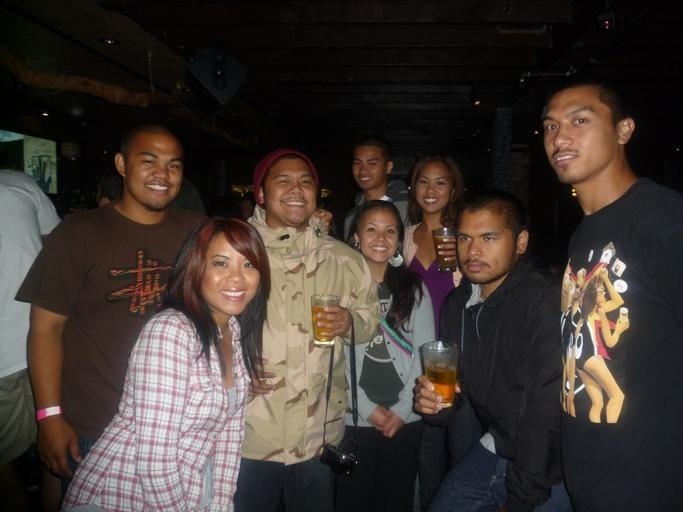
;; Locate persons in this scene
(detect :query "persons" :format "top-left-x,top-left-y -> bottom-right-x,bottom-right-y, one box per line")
334,199 -> 435,512
342,137 -> 412,243
69,174 -> 122,213
0,167 -> 63,512
60,216 -> 270,512
540,83 -> 683,512
14,123 -> 210,498
402,154 -> 464,512
233,148 -> 382,512
414,185 -> 572,512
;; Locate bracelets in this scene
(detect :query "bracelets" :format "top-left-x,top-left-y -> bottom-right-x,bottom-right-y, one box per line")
37,406 -> 64,421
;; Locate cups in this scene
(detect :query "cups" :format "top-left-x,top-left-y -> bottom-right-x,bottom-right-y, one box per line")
619,307 -> 628,322
310,294 -> 338,349
420,340 -> 457,410
431,227 -> 456,273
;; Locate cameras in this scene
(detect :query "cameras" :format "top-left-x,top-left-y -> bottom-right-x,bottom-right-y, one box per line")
322,440 -> 358,475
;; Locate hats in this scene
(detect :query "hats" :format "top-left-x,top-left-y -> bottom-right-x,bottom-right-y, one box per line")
253,148 -> 320,208
